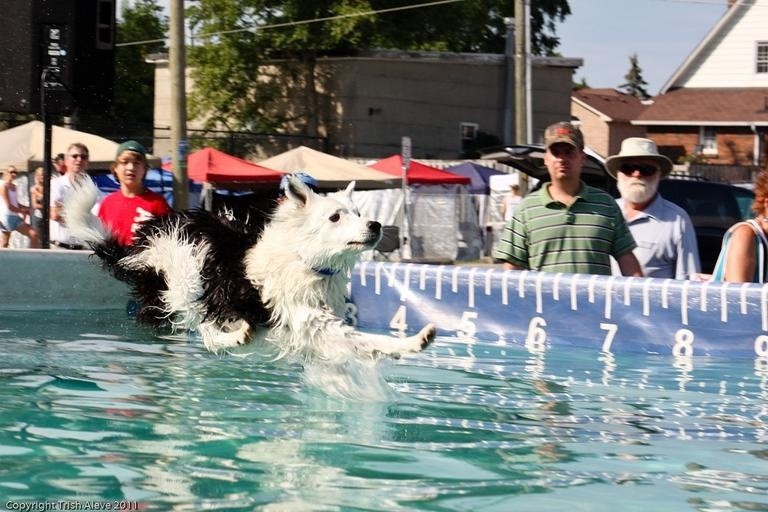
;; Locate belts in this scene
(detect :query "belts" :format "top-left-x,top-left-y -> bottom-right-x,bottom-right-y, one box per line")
49,239 -> 83,249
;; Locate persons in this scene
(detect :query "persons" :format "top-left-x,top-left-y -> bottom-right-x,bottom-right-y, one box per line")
500,183 -> 525,224
0,140 -> 173,252
491,121 -> 643,278
604,136 -> 703,280
711,169 -> 767,284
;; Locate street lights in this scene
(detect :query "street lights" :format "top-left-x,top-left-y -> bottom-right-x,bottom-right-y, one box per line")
37,62 -> 69,249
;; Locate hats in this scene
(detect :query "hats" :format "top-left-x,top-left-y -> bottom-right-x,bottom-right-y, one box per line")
603,137 -> 674,180
544,121 -> 584,150
117,141 -> 146,157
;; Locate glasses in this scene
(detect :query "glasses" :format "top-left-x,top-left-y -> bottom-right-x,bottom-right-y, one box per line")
614,163 -> 661,177
67,154 -> 88,160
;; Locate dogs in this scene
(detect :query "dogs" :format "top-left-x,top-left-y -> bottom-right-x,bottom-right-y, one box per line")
55,171 -> 437,371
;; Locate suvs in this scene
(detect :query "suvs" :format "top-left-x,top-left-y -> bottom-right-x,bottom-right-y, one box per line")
494,141 -> 759,278
